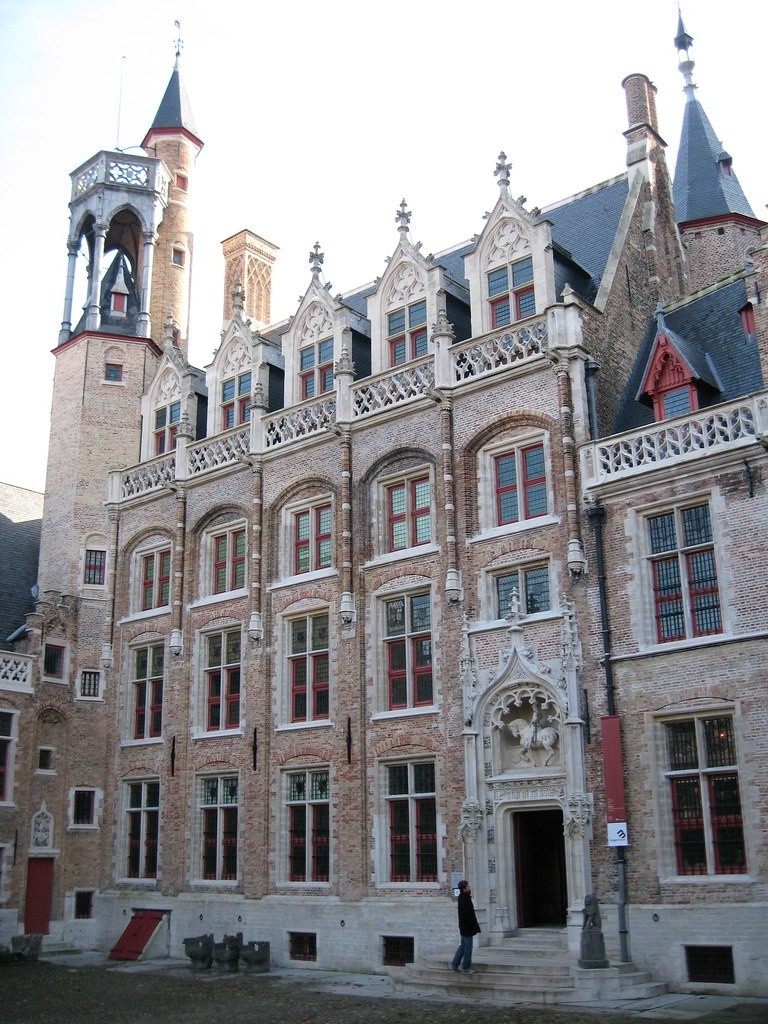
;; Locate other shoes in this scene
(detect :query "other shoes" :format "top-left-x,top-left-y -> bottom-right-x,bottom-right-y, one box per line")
461,969 -> 477,975
452,967 -> 461,973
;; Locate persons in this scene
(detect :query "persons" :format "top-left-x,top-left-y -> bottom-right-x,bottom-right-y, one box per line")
451,881 -> 481,974
